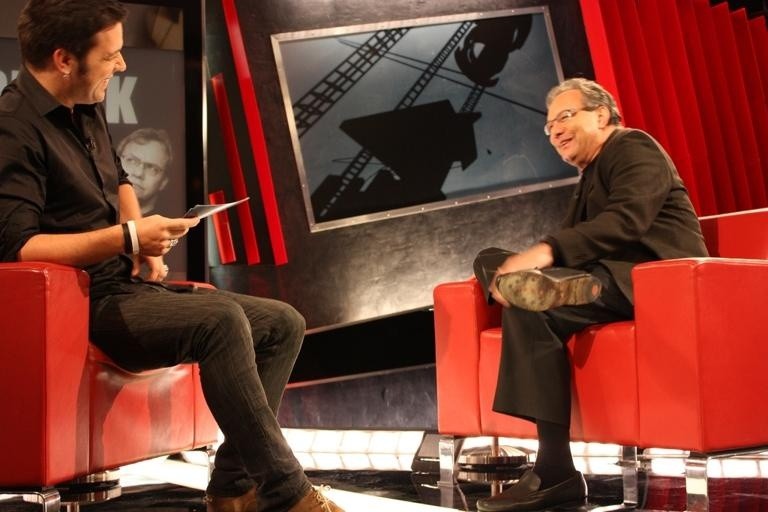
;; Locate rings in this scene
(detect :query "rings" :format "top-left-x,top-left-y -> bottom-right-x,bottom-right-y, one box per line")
170,239 -> 179,246
163,264 -> 169,271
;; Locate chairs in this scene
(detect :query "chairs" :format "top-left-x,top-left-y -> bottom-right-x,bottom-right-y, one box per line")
0,262 -> 217,512
431,208 -> 768,512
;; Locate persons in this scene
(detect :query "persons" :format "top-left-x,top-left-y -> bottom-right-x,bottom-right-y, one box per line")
116,126 -> 173,214
0,0 -> 344,512
474,77 -> 709,512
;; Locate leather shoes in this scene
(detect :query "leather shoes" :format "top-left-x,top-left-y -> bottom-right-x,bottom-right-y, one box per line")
495,267 -> 603,313
476,469 -> 590,511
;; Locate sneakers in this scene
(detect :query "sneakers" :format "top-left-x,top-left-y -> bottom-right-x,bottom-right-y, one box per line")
289,486 -> 344,511
203,483 -> 257,512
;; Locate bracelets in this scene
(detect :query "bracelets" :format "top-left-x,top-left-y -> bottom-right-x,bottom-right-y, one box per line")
123,223 -> 132,255
126,219 -> 139,255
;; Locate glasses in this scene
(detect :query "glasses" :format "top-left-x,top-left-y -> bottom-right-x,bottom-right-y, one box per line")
119,154 -> 164,177
543,107 -> 587,136
